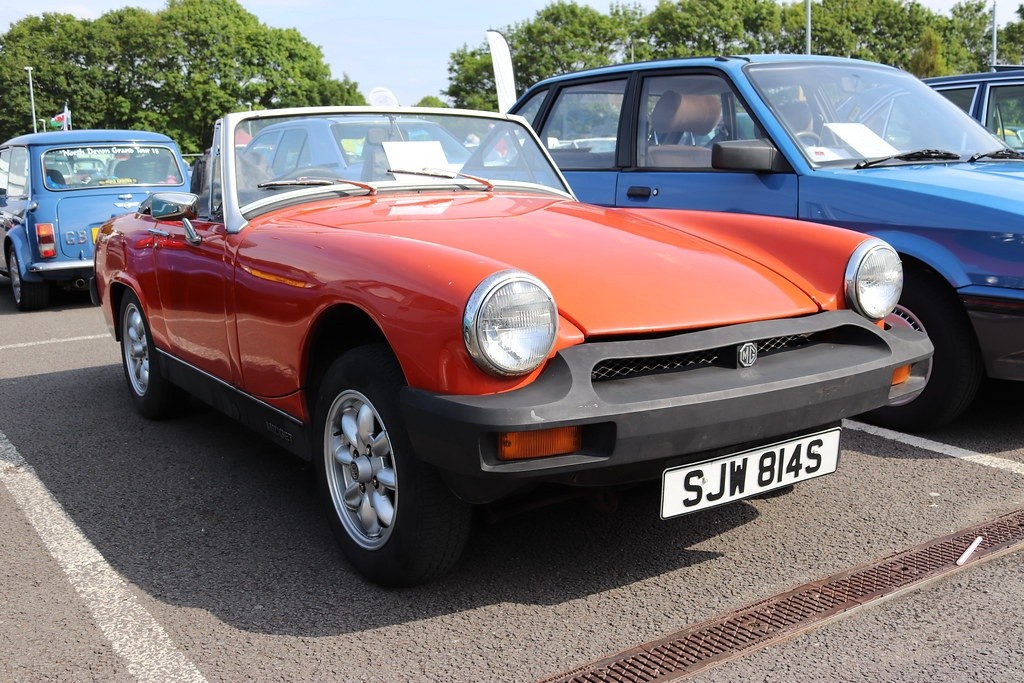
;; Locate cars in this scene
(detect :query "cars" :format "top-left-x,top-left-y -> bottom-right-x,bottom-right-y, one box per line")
558,137 -> 620,152
239,116 -> 473,191
838,63 -> 1023,154
0,130 -> 191,312
455,52 -> 1024,435
88,100 -> 935,586
46,155 -> 195,179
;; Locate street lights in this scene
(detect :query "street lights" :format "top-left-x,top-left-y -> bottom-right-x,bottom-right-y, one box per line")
24,67 -> 38,133
39,118 -> 46,133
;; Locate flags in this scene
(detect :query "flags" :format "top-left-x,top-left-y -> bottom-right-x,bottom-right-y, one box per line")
51,110 -> 71,127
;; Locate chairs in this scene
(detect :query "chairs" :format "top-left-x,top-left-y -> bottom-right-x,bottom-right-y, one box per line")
756,100 -> 814,137
110,157 -> 144,180
641,88 -> 724,169
362,123 -> 412,181
362,126 -> 410,179
188,149 -> 277,195
43,168 -> 66,186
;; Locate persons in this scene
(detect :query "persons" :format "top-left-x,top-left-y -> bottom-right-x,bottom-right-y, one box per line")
488,123 -> 507,156
463,134 -> 499,160
